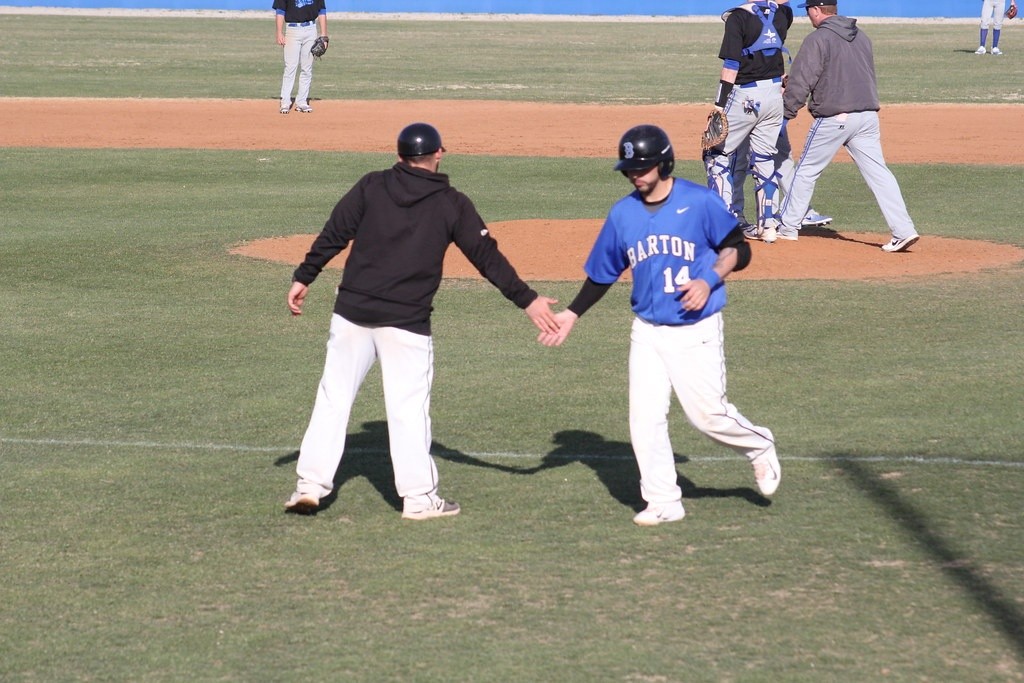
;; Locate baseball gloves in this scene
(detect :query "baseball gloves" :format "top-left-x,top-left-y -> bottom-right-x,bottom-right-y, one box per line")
1005,4 -> 1018,19
700,109 -> 729,151
310,36 -> 329,58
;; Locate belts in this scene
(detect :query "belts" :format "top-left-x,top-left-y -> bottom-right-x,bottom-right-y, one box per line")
740,77 -> 781,88
288,20 -> 316,27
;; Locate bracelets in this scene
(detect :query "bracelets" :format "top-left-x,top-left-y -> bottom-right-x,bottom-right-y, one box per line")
700,270 -> 720,289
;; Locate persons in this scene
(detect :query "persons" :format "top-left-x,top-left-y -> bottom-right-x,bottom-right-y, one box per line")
775,0 -> 920,251
272,0 -> 330,113
285,123 -> 561,519
538,124 -> 781,526
701,0 -> 832,241
975,0 -> 1018,54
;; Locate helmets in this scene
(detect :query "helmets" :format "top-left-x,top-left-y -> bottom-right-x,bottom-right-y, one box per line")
612,125 -> 675,177
397,122 -> 447,155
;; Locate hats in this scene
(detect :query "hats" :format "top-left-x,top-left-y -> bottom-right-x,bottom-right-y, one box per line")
797,0 -> 838,8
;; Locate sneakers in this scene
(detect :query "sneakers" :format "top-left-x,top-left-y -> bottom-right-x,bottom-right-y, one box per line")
633,502 -> 686,526
974,46 -> 986,54
283,490 -> 320,514
751,427 -> 782,497
742,224 -> 777,243
990,46 -> 1002,55
401,497 -> 461,520
279,105 -> 290,114
294,104 -> 313,113
762,217 -> 780,228
881,234 -> 920,252
774,223 -> 799,240
801,212 -> 834,227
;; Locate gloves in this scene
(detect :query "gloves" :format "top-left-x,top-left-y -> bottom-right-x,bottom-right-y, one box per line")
740,96 -> 761,113
